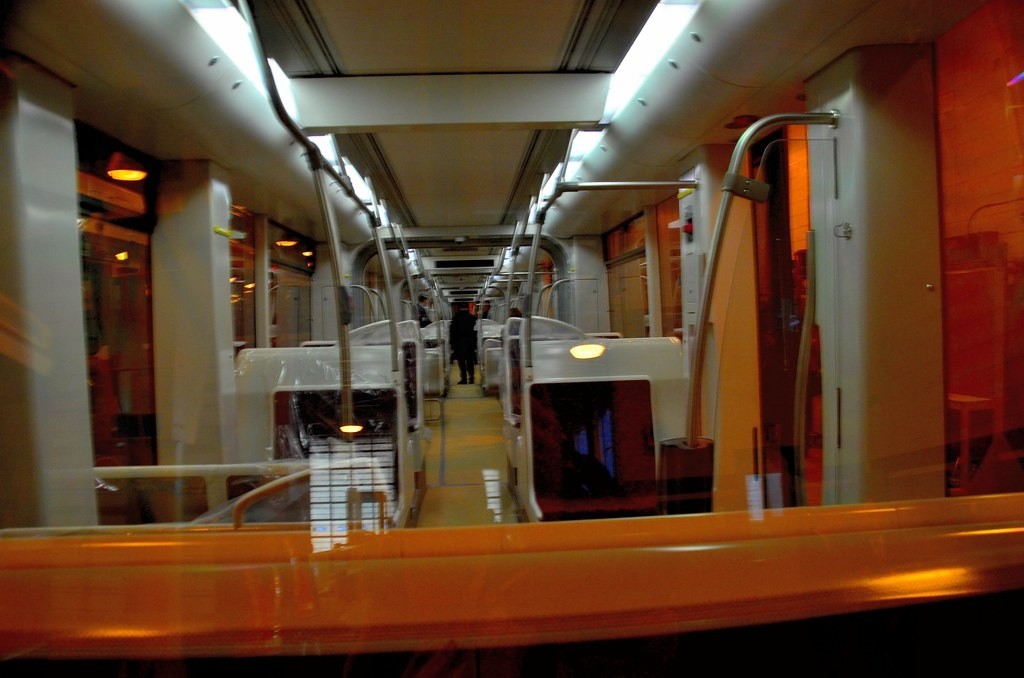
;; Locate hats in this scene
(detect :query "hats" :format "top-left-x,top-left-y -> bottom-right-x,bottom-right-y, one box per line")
418,291 -> 431,298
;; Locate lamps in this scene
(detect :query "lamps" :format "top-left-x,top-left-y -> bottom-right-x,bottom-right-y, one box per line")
276,231 -> 298,249
106,152 -> 148,182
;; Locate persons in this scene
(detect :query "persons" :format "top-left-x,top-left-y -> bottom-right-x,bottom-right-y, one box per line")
793,249 -> 823,447
482,300 -> 490,319
501,308 -> 522,364
449,302 -> 477,384
411,293 -> 437,348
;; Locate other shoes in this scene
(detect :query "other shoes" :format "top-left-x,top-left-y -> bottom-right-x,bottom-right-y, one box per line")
457,378 -> 467,384
469,377 -> 474,384
